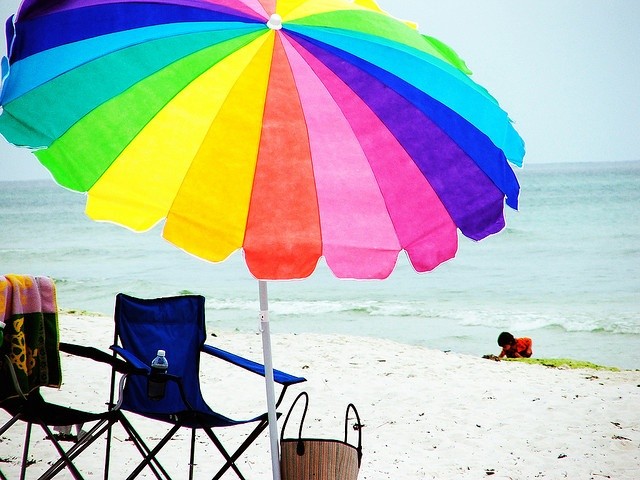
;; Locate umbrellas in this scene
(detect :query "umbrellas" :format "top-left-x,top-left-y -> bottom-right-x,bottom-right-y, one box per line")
0,0 -> 526,480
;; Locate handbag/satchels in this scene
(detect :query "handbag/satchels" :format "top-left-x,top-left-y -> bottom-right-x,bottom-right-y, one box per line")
279,392 -> 362,480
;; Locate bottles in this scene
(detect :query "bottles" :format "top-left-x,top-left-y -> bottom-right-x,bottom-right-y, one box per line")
148,349 -> 168,401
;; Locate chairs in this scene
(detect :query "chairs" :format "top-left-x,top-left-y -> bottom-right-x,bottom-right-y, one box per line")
103,294 -> 307,479
1,274 -> 140,479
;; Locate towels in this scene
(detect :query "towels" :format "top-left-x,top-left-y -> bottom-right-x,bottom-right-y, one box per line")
1,276 -> 61,399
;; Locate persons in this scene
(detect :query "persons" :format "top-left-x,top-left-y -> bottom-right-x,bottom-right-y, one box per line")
497,332 -> 532,358
